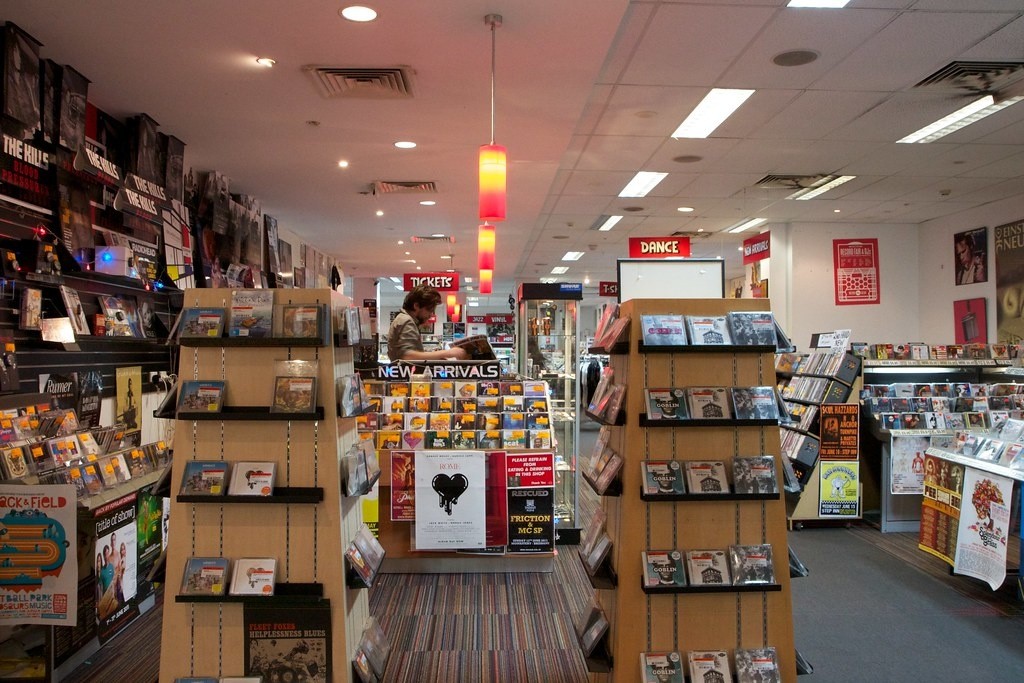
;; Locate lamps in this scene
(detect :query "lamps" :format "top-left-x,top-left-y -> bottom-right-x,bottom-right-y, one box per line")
479,269 -> 497,290
475,222 -> 495,271
451,305 -> 460,322
477,13 -> 505,222
447,254 -> 457,314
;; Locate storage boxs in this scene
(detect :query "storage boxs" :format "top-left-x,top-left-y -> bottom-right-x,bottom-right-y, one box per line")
94,246 -> 134,276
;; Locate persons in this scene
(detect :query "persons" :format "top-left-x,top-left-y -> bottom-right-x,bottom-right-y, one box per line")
388,284 -> 466,363
96,553 -> 103,621
125,378 -> 138,430
929,413 -> 937,428
110,534 -> 125,604
955,236 -> 984,284
111,542 -> 126,598
99,546 -> 115,601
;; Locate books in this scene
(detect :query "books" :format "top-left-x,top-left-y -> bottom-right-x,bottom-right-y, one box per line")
1,290 -> 1024,682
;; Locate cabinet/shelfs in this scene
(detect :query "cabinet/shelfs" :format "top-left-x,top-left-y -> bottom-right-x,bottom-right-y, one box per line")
512,282 -> 583,548
571,299 -> 816,683
862,356 -> 1024,534
157,287 -> 390,683
